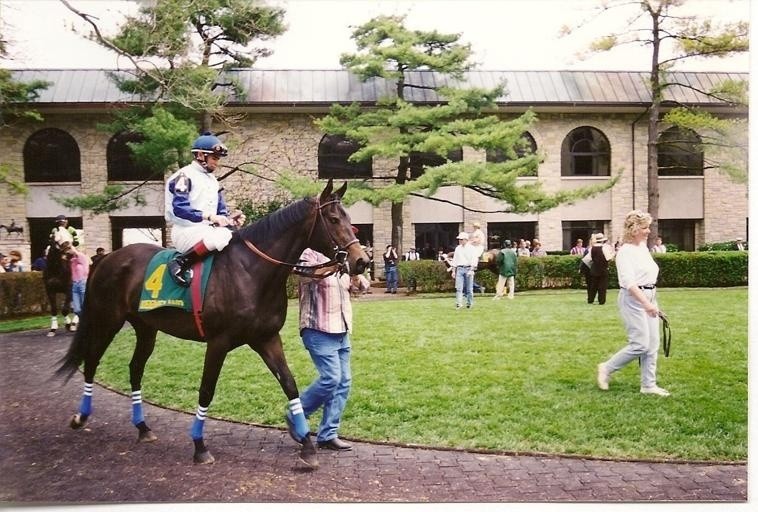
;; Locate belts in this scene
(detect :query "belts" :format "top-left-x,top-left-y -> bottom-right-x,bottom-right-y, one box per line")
638,284 -> 656,289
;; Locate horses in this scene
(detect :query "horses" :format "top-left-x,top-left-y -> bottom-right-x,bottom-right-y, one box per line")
43,177 -> 370,470
440,249 -> 509,296
43,234 -> 72,337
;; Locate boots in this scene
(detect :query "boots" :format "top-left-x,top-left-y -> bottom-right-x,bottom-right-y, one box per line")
169,248 -> 199,288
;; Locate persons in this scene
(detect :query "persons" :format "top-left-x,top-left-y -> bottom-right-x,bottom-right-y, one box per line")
596,209 -> 672,398
284,247 -> 371,452
587,233 -> 613,304
67,242 -> 94,324
650,237 -> 666,254
510,238 -> 547,257
451,231 -> 478,310
570,239 -> 588,255
733,238 -> 744,250
163,134 -> 246,287
1,246 -> 105,273
403,243 -> 455,267
353,239 -> 373,293
494,240 -> 517,297
383,245 -> 399,293
44,215 -> 80,260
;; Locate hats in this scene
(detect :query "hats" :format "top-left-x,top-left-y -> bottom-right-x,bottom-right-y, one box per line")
456,232 -> 469,240
595,233 -> 607,242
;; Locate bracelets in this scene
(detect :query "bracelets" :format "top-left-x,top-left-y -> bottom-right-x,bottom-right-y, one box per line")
207,213 -> 212,221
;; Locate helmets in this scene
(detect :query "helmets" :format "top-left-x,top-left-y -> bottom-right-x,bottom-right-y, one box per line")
55,215 -> 65,223
191,133 -> 228,156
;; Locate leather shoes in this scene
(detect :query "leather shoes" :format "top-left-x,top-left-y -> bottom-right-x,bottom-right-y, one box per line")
318,435 -> 352,450
284,414 -> 302,442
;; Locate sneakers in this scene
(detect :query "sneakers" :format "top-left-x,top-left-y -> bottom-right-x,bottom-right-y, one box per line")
598,362 -> 611,390
640,385 -> 671,396
